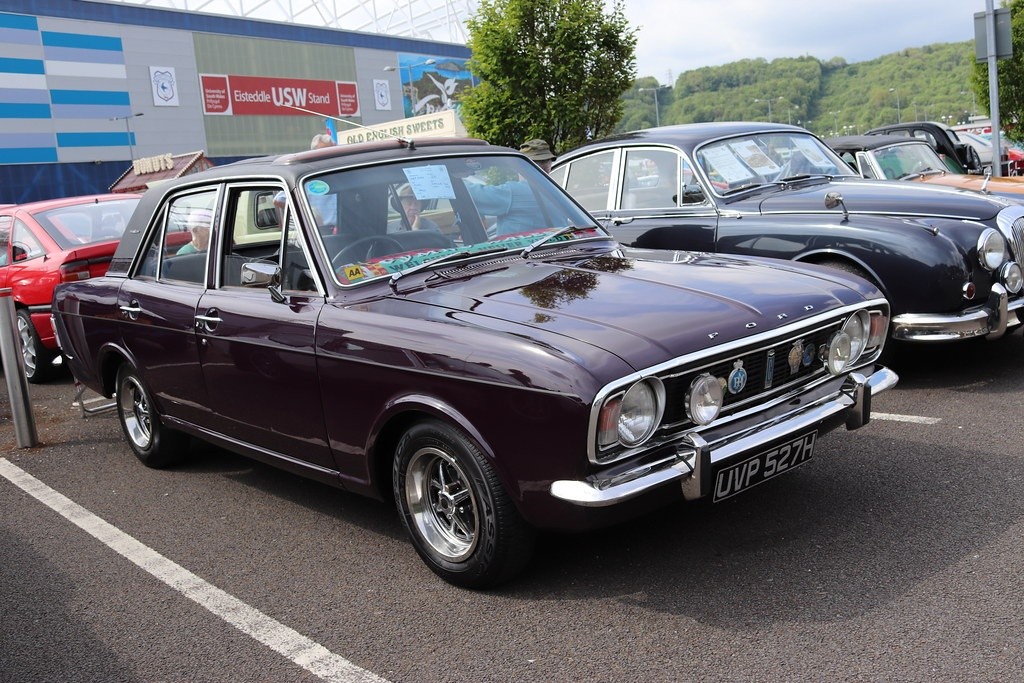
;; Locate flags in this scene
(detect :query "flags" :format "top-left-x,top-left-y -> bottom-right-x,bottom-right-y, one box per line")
325,116 -> 338,145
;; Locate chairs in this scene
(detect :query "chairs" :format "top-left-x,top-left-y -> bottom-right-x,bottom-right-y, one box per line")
342,231 -> 463,266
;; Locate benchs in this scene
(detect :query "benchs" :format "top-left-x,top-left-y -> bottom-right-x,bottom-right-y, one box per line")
164,235 -> 362,280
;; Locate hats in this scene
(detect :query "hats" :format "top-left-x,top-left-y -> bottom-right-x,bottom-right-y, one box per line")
187,210 -> 213,228
390,183 -> 431,213
520,139 -> 557,162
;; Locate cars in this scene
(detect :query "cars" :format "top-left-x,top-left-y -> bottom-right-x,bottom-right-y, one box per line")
0,194 -> 194,383
820,124 -> 1024,195
549,121 -> 1024,343
49,138 -> 900,590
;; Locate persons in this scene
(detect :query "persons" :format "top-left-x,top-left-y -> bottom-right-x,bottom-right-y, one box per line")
177,210 -> 232,254
386,183 -> 439,235
462,138 -> 593,243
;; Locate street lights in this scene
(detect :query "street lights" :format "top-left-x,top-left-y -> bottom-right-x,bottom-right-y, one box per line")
638,85 -> 672,127
385,57 -> 437,117
752,86 -> 978,136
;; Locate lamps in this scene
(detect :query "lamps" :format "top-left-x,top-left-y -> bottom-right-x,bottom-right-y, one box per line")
94,160 -> 102,165
